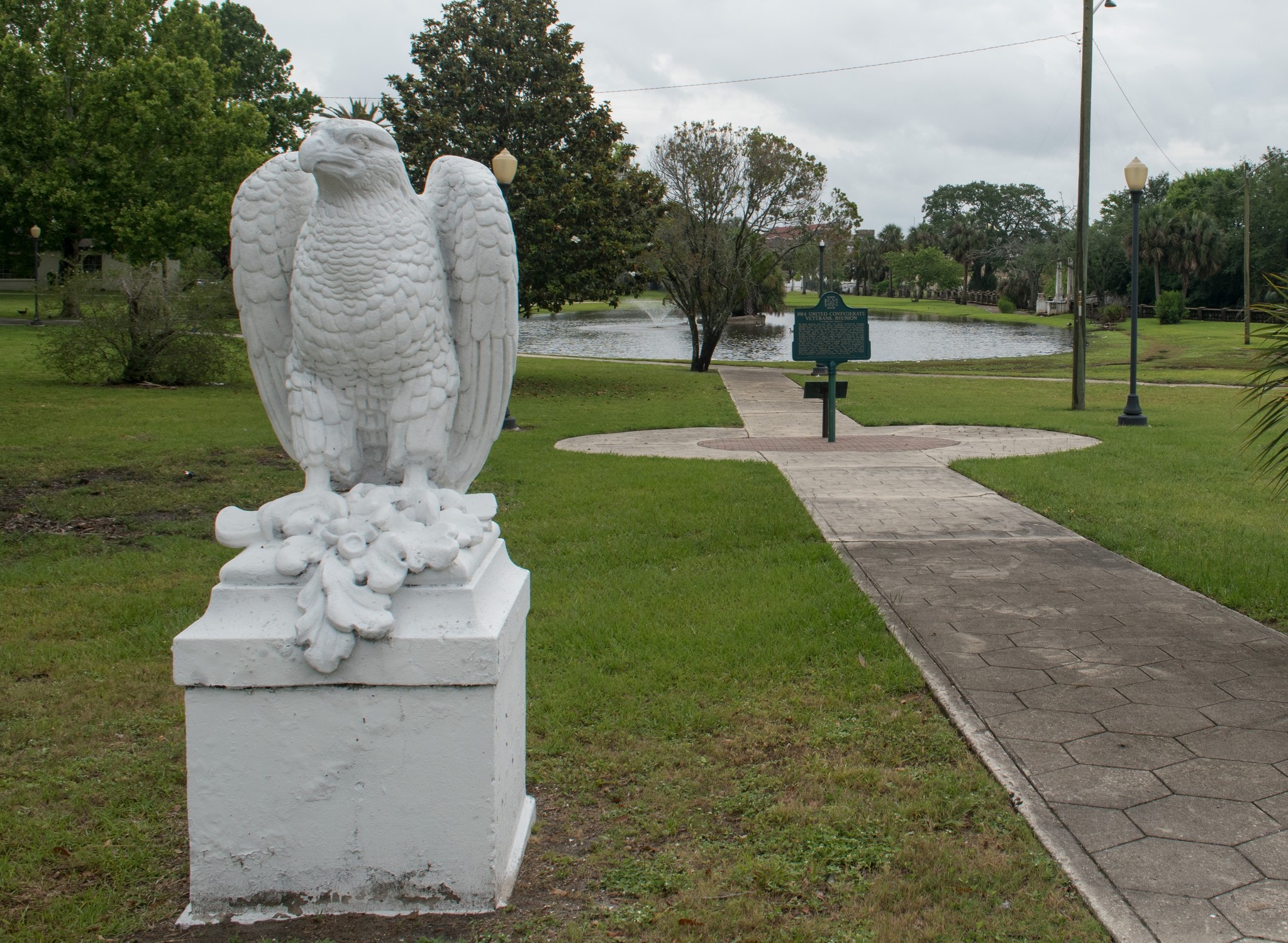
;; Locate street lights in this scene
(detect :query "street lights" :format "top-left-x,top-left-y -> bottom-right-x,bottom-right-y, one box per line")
29,224 -> 41,325
1117,156 -> 1149,427
1070,1 -> 1119,410
490,147 -> 516,429
819,240 -> 825,298
1243,152 -> 1288,345
